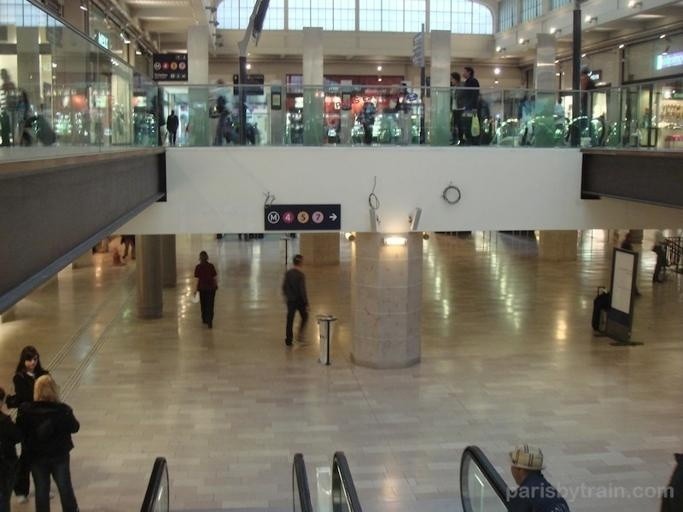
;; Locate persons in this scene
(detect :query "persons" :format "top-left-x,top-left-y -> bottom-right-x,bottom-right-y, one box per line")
0,68 -> 36,149
194,251 -> 218,328
578,65 -> 592,115
282,255 -> 308,345
0,346 -> 79,512
621,233 -> 641,296
167,110 -> 179,146
506,444 -> 571,511
652,231 -> 669,282
120,235 -> 136,259
362,102 -> 375,145
450,65 -> 490,146
209,78 -> 255,145
518,94 -> 564,146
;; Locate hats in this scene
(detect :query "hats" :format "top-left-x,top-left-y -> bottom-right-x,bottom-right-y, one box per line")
505,444 -> 547,470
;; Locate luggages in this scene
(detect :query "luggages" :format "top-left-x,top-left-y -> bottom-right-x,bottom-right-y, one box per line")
591,286 -> 611,334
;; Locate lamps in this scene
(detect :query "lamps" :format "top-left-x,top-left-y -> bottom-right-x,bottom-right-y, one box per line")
382,234 -> 407,250
407,207 -> 423,232
492,0 -> 649,55
367,208 -> 381,233
80,0 -> 137,44
343,232 -> 356,244
205,5 -> 224,50
